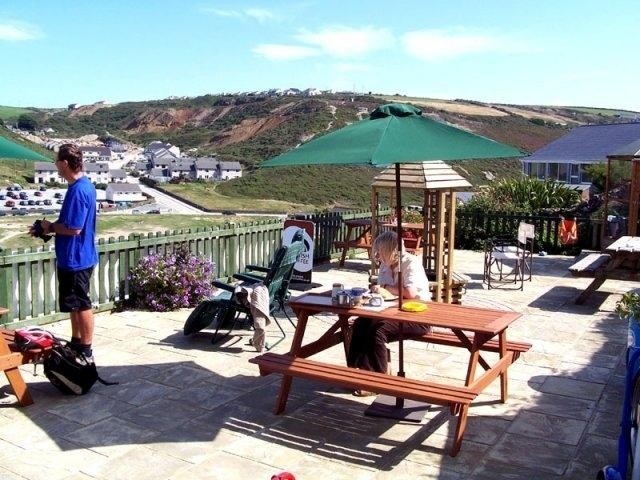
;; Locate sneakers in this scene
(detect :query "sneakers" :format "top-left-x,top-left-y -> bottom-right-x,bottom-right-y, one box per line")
353,389 -> 374,396
75,354 -> 94,365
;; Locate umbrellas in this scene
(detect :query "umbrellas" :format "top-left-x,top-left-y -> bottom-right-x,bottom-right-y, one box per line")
258,103 -> 533,402
1,137 -> 58,164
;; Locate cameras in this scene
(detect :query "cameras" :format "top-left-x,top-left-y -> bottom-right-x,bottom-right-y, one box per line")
31,219 -> 52,243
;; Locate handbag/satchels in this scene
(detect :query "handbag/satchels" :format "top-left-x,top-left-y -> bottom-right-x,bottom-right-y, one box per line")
558,220 -> 579,245
43,347 -> 98,395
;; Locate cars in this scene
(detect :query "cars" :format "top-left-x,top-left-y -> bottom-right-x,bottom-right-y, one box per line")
132,207 -> 162,214
0,181 -> 64,215
222,210 -> 238,217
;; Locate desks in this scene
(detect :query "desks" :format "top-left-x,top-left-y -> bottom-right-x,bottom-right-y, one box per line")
276,287 -> 523,455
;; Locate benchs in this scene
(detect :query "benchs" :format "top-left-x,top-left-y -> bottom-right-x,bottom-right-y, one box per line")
254,349 -> 476,456
346,319 -> 533,370
568,236 -> 640,305
0,306 -> 61,406
333,219 -> 446,271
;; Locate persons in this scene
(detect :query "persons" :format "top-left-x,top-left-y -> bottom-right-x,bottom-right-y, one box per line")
27,142 -> 97,367
347,230 -> 430,398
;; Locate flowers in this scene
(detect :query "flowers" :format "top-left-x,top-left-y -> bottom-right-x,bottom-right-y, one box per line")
125,245 -> 217,314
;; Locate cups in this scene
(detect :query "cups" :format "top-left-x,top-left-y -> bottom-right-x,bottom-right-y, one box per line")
370,298 -> 380,306
352,288 -> 364,303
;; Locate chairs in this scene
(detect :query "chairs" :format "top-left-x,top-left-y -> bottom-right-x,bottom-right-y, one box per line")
183,227 -> 305,351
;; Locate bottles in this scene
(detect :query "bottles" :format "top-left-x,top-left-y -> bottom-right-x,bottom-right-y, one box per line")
336,291 -> 351,306
331,282 -> 345,300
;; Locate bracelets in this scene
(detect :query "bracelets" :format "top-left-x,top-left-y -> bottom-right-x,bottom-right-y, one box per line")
48,223 -> 56,234
383,283 -> 390,289
